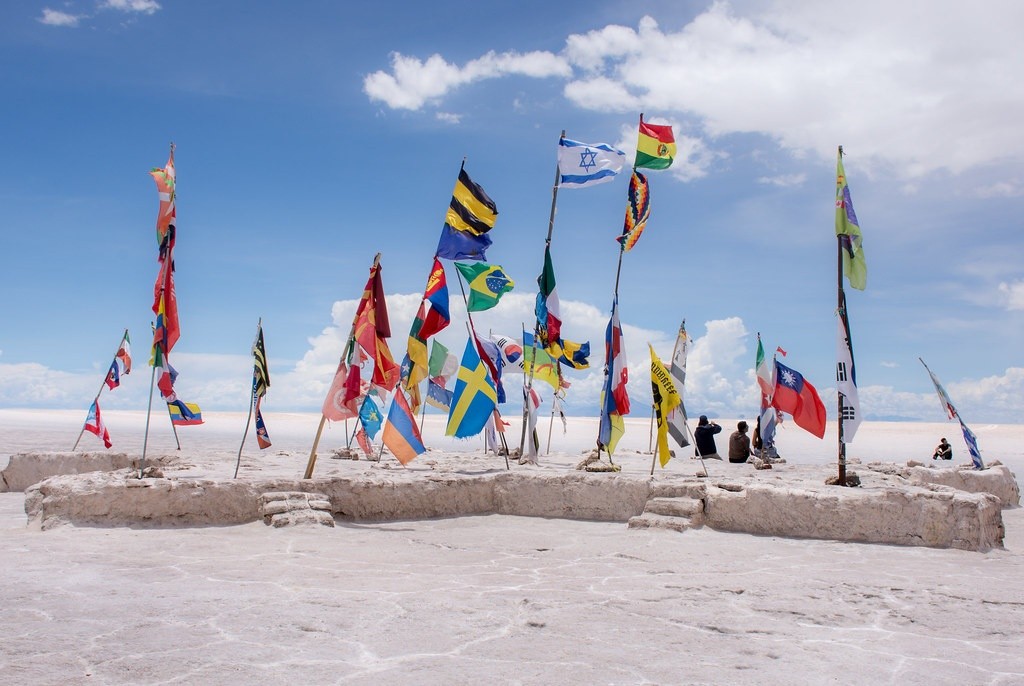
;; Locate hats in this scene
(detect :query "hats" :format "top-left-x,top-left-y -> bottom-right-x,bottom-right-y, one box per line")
700,415 -> 707,420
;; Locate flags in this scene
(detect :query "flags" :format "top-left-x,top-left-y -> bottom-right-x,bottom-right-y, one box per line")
84,401 -> 112,449
117,331 -> 131,374
520,121 -> 984,466
148,150 -> 187,402
250,326 -> 271,396
428,340 -> 458,388
322,263 -> 427,468
426,379 -> 454,414
105,359 -> 119,391
435,168 -> 497,261
417,259 -> 450,340
455,263 -> 515,312
445,338 -> 497,438
473,331 -> 525,454
169,404 -> 207,426
252,377 -> 272,449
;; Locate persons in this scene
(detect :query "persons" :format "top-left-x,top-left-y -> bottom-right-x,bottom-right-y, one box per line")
694,415 -> 722,460
728,421 -> 751,463
933,438 -> 952,460
752,415 -> 764,454
